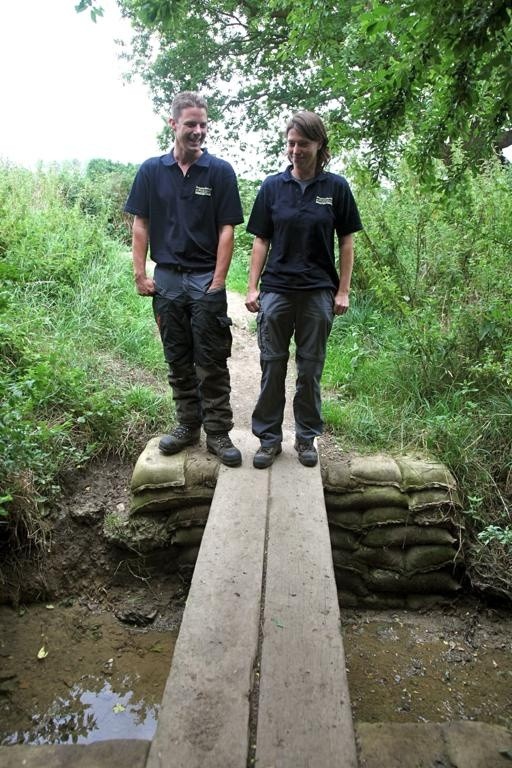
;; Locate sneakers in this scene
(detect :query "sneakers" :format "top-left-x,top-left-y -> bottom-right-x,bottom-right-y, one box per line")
206,434 -> 242,466
293,441 -> 318,467
158,423 -> 201,454
253,443 -> 282,469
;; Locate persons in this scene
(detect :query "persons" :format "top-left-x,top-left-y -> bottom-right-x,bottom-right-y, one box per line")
123,92 -> 246,468
244,111 -> 362,471
